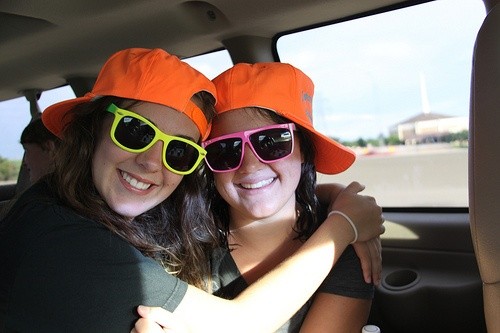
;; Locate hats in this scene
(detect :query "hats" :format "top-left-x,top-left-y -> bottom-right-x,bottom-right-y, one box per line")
41,48 -> 218,141
211,62 -> 357,175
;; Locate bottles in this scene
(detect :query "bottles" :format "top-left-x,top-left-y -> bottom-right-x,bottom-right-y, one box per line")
361,324 -> 380,333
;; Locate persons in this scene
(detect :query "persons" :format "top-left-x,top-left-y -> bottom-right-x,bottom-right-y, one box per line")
0,118 -> 64,225
130,62 -> 381,333
0,47 -> 387,333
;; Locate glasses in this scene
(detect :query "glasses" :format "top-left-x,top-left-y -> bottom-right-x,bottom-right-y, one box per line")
107,103 -> 208,175
198,121 -> 299,174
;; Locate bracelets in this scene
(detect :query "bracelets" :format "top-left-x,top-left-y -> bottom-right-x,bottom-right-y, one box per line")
328,211 -> 358,245
